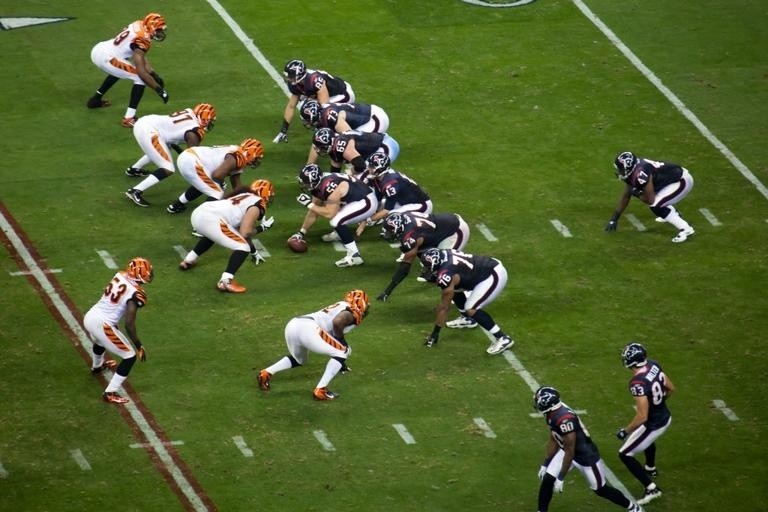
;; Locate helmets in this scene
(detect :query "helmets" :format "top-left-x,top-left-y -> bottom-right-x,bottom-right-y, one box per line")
240,137 -> 264,169
250,178 -> 275,207
378,213 -> 409,242
419,246 -> 445,279
363,151 -> 391,178
298,99 -> 324,128
295,162 -> 323,194
342,289 -> 371,321
311,128 -> 338,158
126,257 -> 155,285
621,339 -> 649,369
613,149 -> 639,183
194,102 -> 217,132
282,60 -> 308,87
532,387 -> 560,413
143,12 -> 166,42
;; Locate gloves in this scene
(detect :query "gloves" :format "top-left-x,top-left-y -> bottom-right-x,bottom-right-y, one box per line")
136,345 -> 148,363
294,193 -> 314,208
554,473 -> 565,494
616,428 -> 629,440
603,216 -> 618,233
338,363 -> 353,375
631,187 -> 644,198
537,464 -> 548,481
289,228 -> 308,241
251,249 -> 267,266
425,330 -> 439,348
272,127 -> 289,144
344,343 -> 353,356
259,213 -> 275,231
157,87 -> 169,104
152,73 -> 164,88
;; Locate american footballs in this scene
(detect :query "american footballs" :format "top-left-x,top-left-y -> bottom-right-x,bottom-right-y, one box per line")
288,238 -> 307,252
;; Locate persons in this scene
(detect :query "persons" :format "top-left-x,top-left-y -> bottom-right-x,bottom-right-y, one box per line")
176,177 -> 273,293
161,138 -> 263,212
603,146 -> 698,246
533,386 -> 644,511
83,255 -> 151,405
85,12 -> 170,131
613,342 -> 676,503
120,102 -> 214,207
252,291 -> 373,400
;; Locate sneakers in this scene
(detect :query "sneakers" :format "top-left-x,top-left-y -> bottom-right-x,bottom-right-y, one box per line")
629,462 -> 665,512
191,229 -> 203,238
120,115 -> 139,129
445,314 -> 480,329
256,368 -> 273,392
167,200 -> 187,214
124,187 -> 151,208
179,257 -> 197,271
311,386 -> 341,401
654,213 -> 695,243
90,359 -> 117,377
321,220 -> 430,303
102,389 -> 131,405
85,98 -> 112,108
216,278 -> 247,293
486,334 -> 515,356
125,165 -> 153,177
